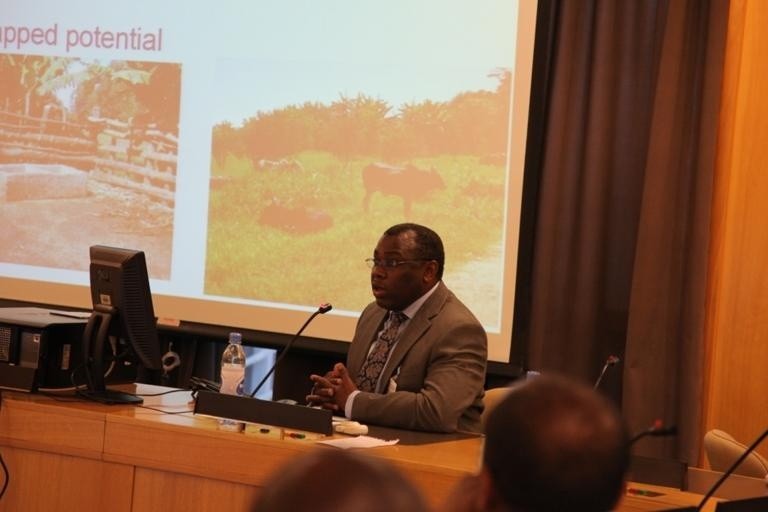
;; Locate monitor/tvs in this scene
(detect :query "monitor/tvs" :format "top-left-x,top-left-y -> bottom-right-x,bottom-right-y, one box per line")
76,245 -> 164,404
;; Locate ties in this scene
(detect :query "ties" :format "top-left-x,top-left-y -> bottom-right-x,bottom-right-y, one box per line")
355,311 -> 409,393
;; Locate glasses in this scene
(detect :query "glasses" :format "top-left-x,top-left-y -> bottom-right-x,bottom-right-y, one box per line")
365,258 -> 417,271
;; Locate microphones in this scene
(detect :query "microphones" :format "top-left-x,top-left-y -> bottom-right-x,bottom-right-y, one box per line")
249,302 -> 332,397
593,355 -> 619,389
630,425 -> 676,445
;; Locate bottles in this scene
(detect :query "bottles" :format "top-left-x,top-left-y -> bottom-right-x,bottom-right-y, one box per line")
220,333 -> 245,396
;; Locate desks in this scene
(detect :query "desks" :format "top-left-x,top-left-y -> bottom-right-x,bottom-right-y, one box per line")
0,382 -> 768,512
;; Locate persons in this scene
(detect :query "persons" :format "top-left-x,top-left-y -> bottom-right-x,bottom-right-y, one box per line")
306,224 -> 487,436
441,376 -> 632,512
251,448 -> 426,512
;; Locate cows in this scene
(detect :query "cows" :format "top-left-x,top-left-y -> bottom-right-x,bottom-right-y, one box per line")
360,162 -> 447,219
258,198 -> 333,234
